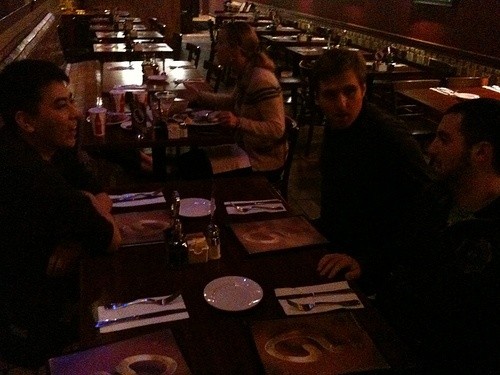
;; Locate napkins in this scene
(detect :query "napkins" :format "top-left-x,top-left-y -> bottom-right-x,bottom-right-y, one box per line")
224,199 -> 287,214
109,191 -> 167,207
482,85 -> 500,93
98,293 -> 190,333
274,280 -> 364,316
430,87 -> 457,96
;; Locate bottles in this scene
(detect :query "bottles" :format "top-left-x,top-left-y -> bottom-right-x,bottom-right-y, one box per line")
166,220 -> 189,268
206,223 -> 222,260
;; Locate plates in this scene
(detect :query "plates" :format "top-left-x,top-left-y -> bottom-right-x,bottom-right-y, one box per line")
204,275 -> 263,311
455,93 -> 480,100
174,109 -> 225,125
171,197 -> 216,217
120,121 -> 153,129
86,112 -> 129,124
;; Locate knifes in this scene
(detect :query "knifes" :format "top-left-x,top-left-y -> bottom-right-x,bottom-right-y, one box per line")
96,307 -> 188,328
223,199 -> 281,207
271,288 -> 358,302
112,195 -> 164,202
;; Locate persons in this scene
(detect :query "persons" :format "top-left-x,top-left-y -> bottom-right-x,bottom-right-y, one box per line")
0,59 -> 121,368
317,48 -> 500,375
183,22 -> 291,185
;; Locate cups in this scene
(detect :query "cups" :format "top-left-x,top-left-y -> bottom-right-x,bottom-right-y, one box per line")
109,88 -> 176,125
88,107 -> 106,136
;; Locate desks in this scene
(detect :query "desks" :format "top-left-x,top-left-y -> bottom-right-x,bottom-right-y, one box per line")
66,10 -> 500,374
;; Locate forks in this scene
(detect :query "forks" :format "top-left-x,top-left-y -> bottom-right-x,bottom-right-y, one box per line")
116,188 -> 164,199
285,298 -> 361,311
105,293 -> 181,312
231,203 -> 283,213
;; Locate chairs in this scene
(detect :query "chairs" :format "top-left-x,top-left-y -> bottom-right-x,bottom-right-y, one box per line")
298,59 -> 326,157
266,45 -> 301,118
276,115 -> 299,202
208,18 -> 217,61
148,17 -> 158,30
204,60 -> 221,93
172,33 -> 182,61
186,42 -> 201,69
158,23 -> 166,43
